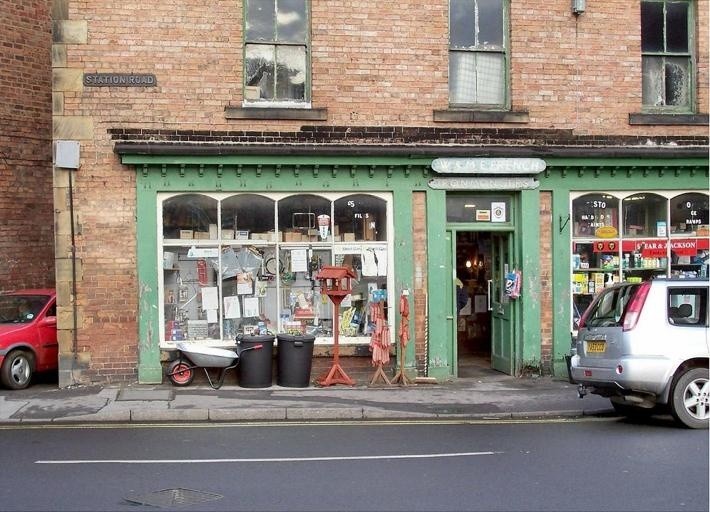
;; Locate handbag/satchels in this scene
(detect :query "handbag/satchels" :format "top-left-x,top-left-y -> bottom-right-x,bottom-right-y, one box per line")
511,272 -> 522,299
504,272 -> 515,296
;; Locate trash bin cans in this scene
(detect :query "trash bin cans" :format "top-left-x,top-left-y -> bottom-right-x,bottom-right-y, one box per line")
277,330 -> 315,387
235,333 -> 275,388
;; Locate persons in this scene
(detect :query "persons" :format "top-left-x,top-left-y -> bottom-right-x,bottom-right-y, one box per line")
457,278 -> 468,321
16,297 -> 31,321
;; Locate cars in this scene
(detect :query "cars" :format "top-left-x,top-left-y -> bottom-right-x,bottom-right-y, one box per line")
1,289 -> 57,390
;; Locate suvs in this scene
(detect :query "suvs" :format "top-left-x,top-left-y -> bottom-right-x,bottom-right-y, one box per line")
570,279 -> 709,431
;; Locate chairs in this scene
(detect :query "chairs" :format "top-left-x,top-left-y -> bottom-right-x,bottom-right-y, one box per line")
670,304 -> 697,324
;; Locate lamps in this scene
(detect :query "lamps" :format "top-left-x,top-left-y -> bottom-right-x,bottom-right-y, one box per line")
465,260 -> 473,273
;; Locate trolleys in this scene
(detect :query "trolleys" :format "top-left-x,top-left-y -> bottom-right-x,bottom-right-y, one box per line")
167,343 -> 262,390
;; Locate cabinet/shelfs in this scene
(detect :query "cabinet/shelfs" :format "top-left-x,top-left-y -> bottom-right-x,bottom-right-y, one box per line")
569,189 -> 710,337
156,191 -> 395,350
